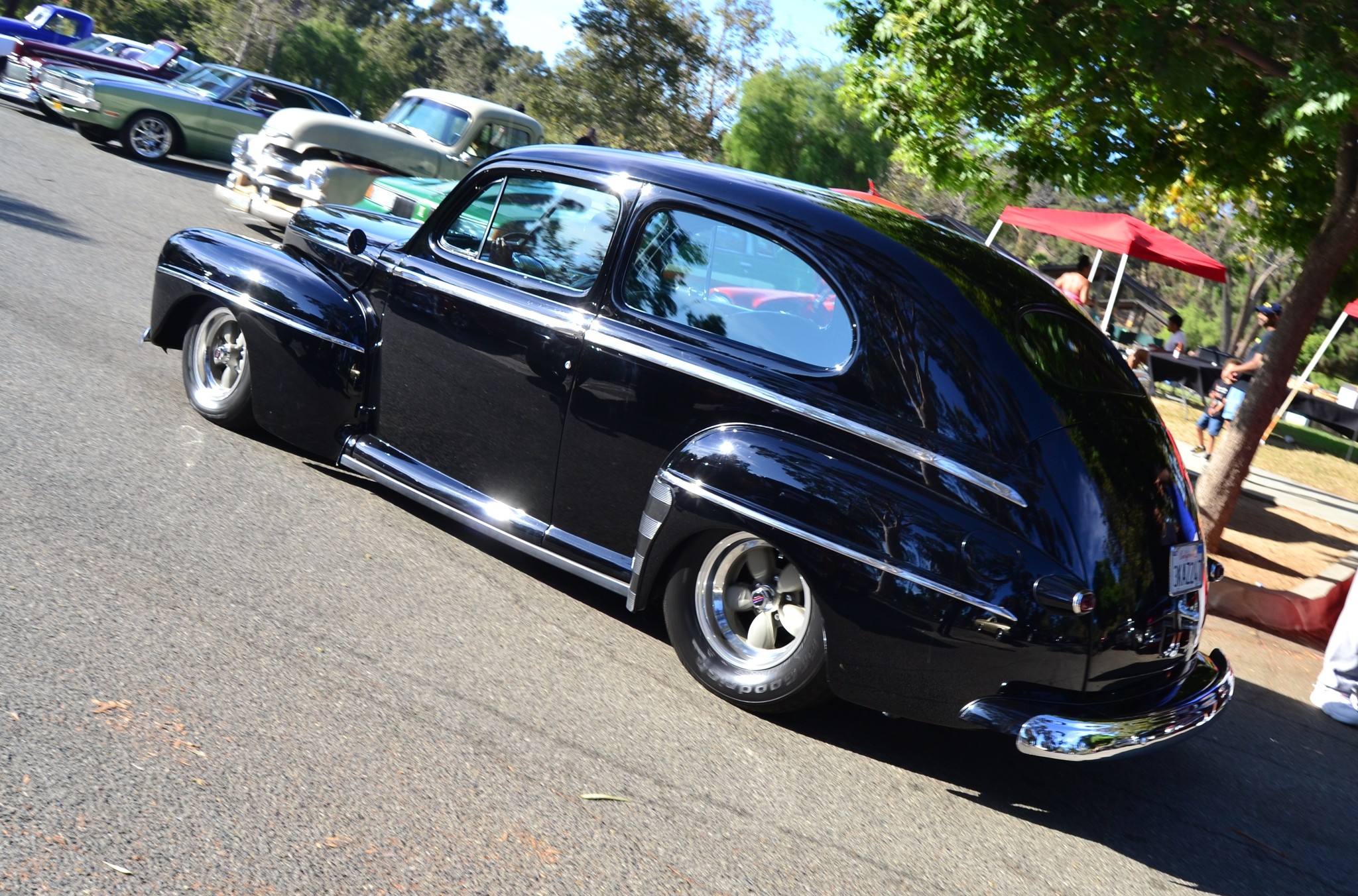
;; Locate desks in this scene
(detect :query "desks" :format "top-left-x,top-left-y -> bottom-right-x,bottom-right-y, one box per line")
1277,388 -> 1358,461
1147,351 -> 1223,407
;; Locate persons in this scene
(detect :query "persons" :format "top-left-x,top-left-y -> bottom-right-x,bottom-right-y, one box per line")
1126,313 -> 1187,370
575,127 -> 595,145
1054,254 -> 1096,306
488,209 -> 618,290
1190,358 -> 1243,462
1220,302 -> 1285,429
489,102 -> 526,149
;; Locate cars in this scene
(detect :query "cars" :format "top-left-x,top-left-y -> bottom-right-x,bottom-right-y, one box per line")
214,88 -> 547,233
31,58 -> 360,166
139,141 -> 1238,765
0,2 -> 283,129
348,165 -> 837,325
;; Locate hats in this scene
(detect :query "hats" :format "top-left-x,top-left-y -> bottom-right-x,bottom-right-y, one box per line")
1253,301 -> 1284,316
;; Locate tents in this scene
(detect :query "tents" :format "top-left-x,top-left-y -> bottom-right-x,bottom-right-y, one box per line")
985,204 -> 1229,353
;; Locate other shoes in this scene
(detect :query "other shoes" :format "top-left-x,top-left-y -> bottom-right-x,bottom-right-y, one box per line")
1311,680 -> 1358,725
1191,445 -> 1206,456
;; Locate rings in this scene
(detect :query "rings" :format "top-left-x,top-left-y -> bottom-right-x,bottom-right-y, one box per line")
496,246 -> 503,250
